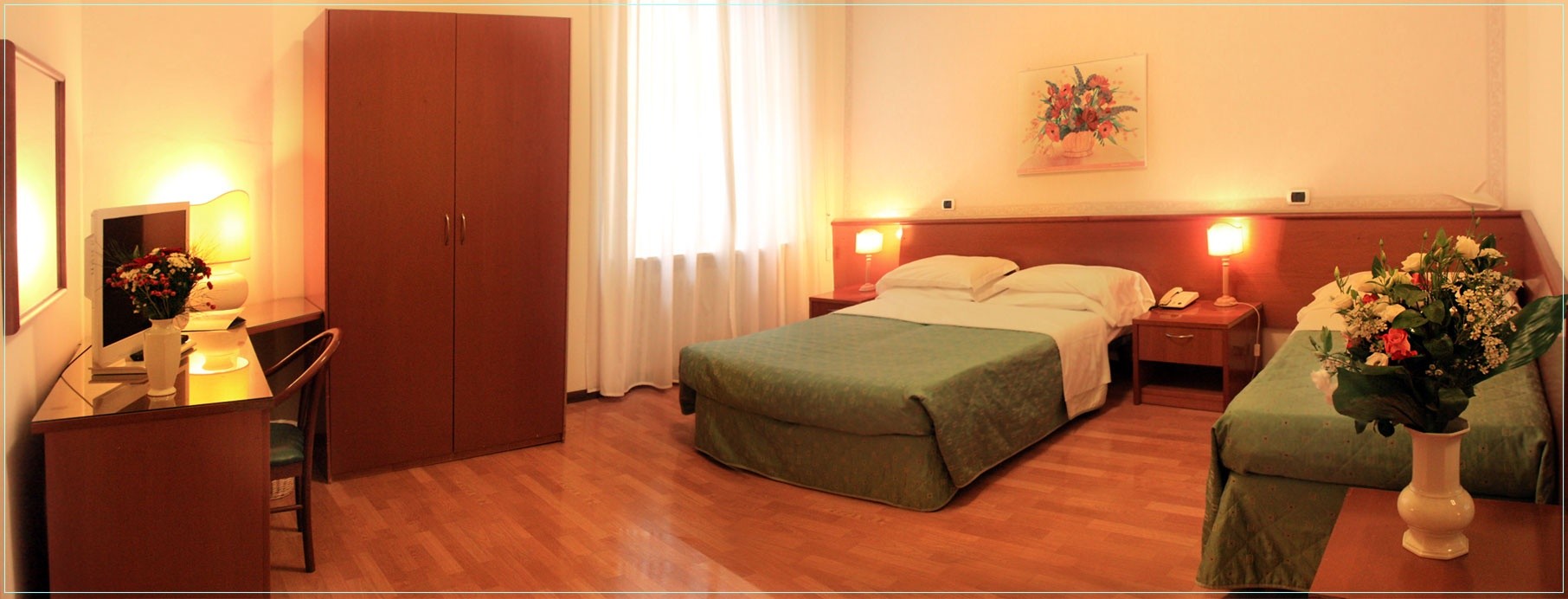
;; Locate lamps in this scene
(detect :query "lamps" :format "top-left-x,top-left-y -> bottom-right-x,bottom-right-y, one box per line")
1207,222 -> 1245,307
190,189 -> 253,310
854,229 -> 883,292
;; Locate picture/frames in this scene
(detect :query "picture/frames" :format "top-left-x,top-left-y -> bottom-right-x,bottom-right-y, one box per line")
0,37 -> 67,335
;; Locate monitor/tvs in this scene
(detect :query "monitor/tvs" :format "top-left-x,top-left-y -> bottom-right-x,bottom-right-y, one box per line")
84,200 -> 192,374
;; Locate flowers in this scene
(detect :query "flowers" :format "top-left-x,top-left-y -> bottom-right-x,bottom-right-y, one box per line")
89,236 -> 218,328
1301,205 -> 1568,438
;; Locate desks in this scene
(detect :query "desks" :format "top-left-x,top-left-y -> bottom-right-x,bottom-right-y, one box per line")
1308,485 -> 1568,598
30,295 -> 324,599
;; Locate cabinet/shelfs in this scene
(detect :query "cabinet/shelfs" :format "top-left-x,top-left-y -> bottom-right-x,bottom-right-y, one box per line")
302,8 -> 574,485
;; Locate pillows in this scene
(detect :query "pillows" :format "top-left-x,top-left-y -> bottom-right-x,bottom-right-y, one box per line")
874,253 -> 1019,292
1297,269 -> 1524,317
881,277 -> 1010,303
996,264 -> 1156,328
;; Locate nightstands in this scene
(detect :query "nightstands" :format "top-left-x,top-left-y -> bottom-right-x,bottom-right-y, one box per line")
1132,298 -> 1263,409
809,290 -> 879,319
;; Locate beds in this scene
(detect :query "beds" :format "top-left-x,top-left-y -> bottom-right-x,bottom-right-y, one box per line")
677,302 -> 1133,513
1192,316 -> 1554,591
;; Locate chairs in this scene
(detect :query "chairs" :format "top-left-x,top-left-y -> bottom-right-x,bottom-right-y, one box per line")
264,327 -> 343,573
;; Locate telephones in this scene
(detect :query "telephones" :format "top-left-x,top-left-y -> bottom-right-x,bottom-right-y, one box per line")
1157,287 -> 1199,308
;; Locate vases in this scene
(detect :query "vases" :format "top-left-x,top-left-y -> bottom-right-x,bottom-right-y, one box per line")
1394,416 -> 1479,558
141,319 -> 182,397
